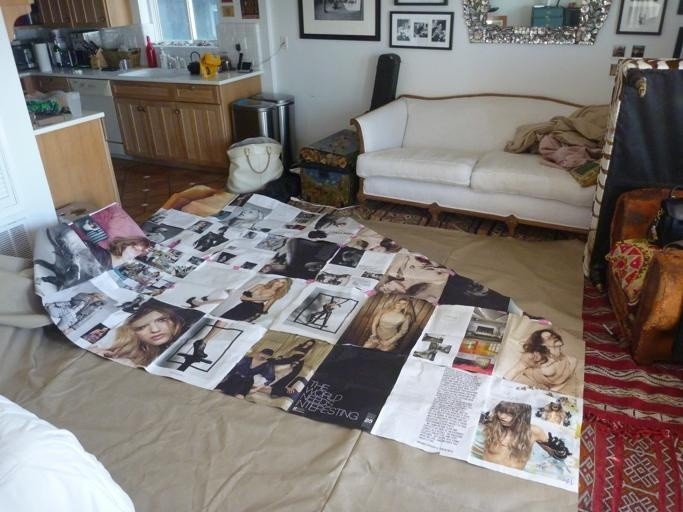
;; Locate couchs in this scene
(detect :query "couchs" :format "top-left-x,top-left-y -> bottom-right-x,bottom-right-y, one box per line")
349,94 -> 595,238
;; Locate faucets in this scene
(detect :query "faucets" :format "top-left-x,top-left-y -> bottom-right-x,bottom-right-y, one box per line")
165,54 -> 181,69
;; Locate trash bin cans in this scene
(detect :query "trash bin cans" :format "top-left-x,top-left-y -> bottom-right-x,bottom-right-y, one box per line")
230,91 -> 298,165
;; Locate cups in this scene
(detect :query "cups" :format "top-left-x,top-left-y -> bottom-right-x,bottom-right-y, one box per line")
66,92 -> 82,118
120,59 -> 128,73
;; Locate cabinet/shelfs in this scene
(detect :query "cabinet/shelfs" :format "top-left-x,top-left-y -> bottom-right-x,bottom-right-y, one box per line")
531,7 -> 581,27
19,76 -> 130,159
111,81 -> 229,176
37,1 -> 133,27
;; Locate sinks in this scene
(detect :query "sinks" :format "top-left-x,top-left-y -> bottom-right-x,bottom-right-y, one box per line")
118,67 -> 182,79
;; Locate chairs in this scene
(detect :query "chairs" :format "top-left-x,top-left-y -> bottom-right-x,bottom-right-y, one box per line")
608,188 -> 682,364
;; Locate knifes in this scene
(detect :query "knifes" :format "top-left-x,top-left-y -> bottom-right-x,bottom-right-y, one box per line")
82,40 -> 98,56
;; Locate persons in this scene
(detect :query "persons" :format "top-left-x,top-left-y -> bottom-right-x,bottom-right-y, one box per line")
432,22 -> 445,40
28,210 -> 579,469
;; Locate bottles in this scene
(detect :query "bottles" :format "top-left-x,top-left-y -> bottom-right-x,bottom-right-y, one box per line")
159,48 -> 168,69
146,36 -> 157,67
52,40 -> 65,68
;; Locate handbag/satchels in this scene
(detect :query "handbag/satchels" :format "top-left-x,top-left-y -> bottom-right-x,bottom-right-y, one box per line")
226,137 -> 285,194
648,185 -> 683,245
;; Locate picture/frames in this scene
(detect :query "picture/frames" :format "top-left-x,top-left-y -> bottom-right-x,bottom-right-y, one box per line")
298,0 -> 454,50
616,0 -> 668,36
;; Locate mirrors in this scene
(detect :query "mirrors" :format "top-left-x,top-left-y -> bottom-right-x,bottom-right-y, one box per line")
462,0 -> 612,46
